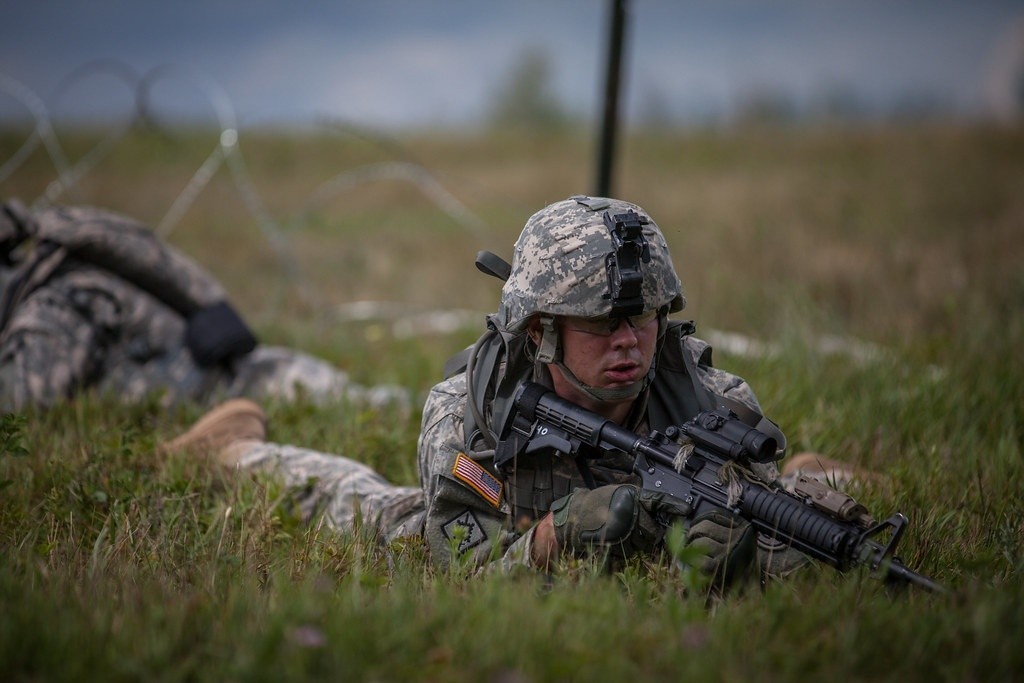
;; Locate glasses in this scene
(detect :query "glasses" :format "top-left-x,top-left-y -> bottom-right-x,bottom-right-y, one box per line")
555,305 -> 669,335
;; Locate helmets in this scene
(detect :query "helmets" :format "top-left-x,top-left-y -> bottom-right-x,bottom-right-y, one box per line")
493,195 -> 686,334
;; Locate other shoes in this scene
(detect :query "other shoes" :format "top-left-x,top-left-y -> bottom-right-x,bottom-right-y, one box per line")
153,398 -> 268,482
785,452 -> 897,492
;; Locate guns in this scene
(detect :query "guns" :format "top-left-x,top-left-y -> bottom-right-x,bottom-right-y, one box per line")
464,379 -> 945,604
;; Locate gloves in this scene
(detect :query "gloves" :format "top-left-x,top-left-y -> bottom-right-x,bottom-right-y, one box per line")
550,483 -> 694,561
678,508 -> 758,599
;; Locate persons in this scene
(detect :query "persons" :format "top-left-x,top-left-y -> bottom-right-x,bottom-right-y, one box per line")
0,196 -> 425,424
163,196 -> 893,611
